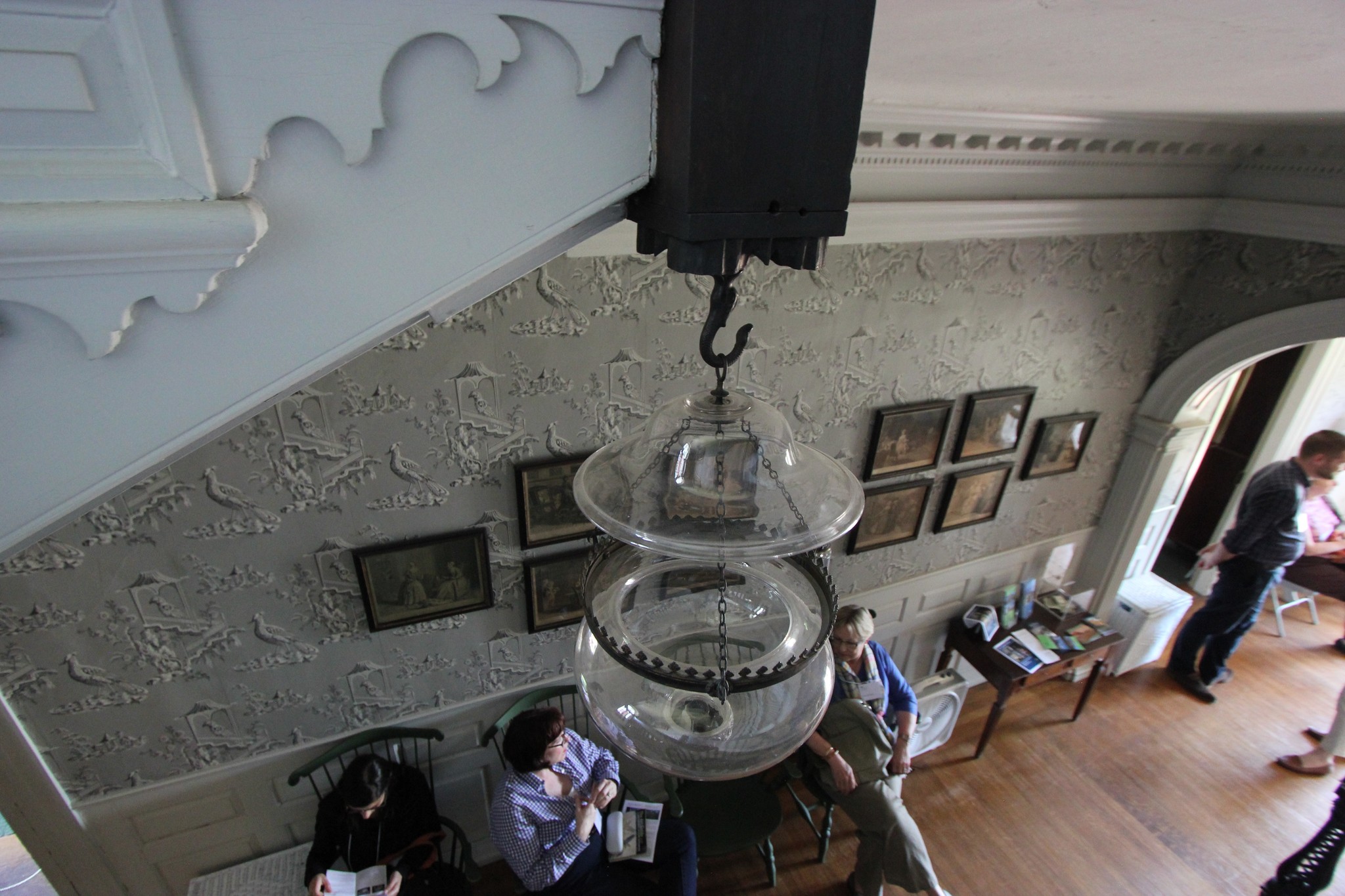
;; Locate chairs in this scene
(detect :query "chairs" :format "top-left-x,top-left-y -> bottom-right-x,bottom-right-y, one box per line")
288,631 -> 836,889
1270,581 -> 1319,637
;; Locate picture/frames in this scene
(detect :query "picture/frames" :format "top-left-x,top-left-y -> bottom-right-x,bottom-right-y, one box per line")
1018,410 -> 1104,483
513,450 -> 607,553
658,524 -> 749,600
952,386 -> 1038,464
862,398 -> 954,481
933,459 -> 1015,535
664,440 -> 758,521
846,477 -> 933,554
350,526 -> 494,634
523,542 -> 637,636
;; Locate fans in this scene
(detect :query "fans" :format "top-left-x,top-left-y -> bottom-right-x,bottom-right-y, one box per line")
893,669 -> 970,759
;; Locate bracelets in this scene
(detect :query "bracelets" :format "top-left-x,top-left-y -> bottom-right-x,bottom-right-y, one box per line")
824,747 -> 839,761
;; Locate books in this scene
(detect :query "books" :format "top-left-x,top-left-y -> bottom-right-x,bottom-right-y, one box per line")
608,809 -> 647,863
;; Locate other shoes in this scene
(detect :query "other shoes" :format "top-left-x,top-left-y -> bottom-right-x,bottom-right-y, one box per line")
1277,754 -> 1333,776
1170,670 -> 1216,702
1206,669 -> 1233,686
1308,726 -> 1327,739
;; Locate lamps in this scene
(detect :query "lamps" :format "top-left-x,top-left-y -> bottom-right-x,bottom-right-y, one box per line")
571,353 -> 868,778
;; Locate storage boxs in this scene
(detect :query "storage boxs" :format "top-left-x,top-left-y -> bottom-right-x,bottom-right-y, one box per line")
1035,569 -> 1096,630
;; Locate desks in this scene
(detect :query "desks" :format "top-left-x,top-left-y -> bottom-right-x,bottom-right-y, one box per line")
187,839 -> 350,896
936,601 -> 1126,757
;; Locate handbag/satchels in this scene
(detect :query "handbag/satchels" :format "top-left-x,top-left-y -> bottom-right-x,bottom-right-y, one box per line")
819,698 -> 897,792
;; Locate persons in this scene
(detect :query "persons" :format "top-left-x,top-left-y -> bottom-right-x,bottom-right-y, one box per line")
303,754 -> 443,896
786,604 -> 952,896
1166,430 -> 1345,775
487,708 -> 698,896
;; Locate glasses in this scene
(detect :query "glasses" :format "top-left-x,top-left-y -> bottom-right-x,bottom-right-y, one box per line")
829,633 -> 861,649
547,731 -> 566,750
346,792 -> 388,814
